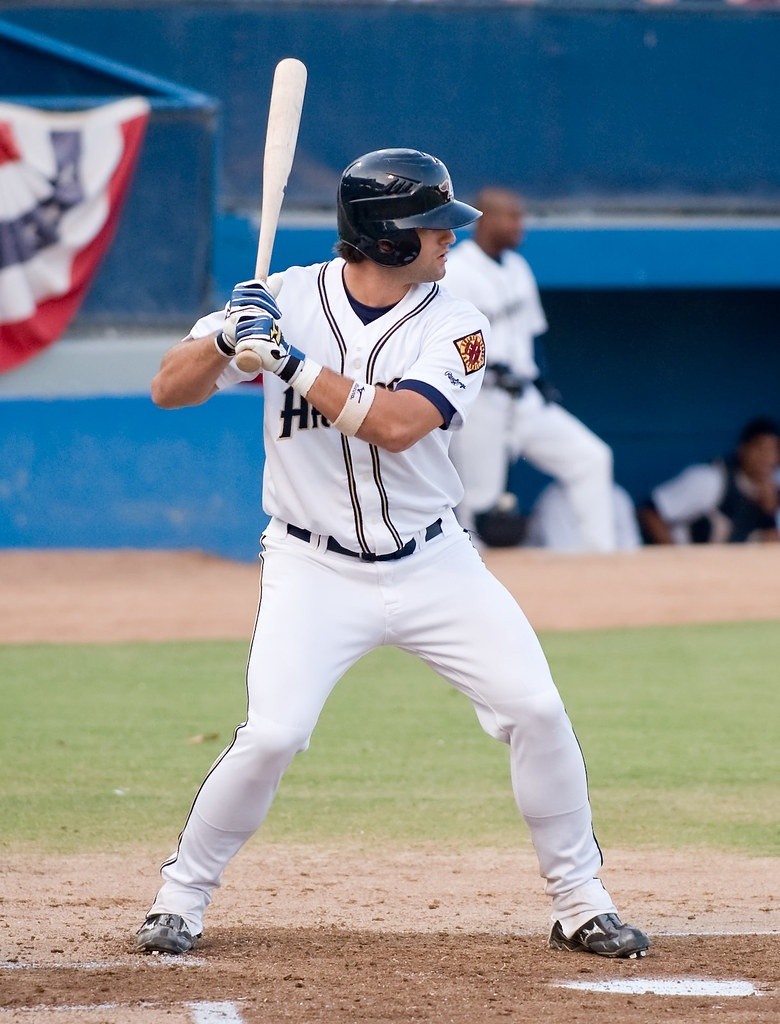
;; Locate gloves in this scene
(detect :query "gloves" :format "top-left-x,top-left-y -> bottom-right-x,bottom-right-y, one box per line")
235,315 -> 322,399
216,279 -> 278,370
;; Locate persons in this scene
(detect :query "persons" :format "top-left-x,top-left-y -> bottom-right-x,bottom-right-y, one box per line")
442,186 -> 780,553
132,152 -> 651,962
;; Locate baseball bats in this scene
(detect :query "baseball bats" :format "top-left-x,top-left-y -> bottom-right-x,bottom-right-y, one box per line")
238,56 -> 307,374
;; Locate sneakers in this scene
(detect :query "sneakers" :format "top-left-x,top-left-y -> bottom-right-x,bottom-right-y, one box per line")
133,913 -> 198,955
548,911 -> 650,960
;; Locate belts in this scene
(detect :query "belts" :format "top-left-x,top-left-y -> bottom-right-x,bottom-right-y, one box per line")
287,518 -> 443,562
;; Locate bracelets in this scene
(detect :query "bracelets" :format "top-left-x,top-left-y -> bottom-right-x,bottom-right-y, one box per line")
328,381 -> 376,439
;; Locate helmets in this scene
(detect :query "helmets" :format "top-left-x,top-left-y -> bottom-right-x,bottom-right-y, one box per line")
335,149 -> 483,267
